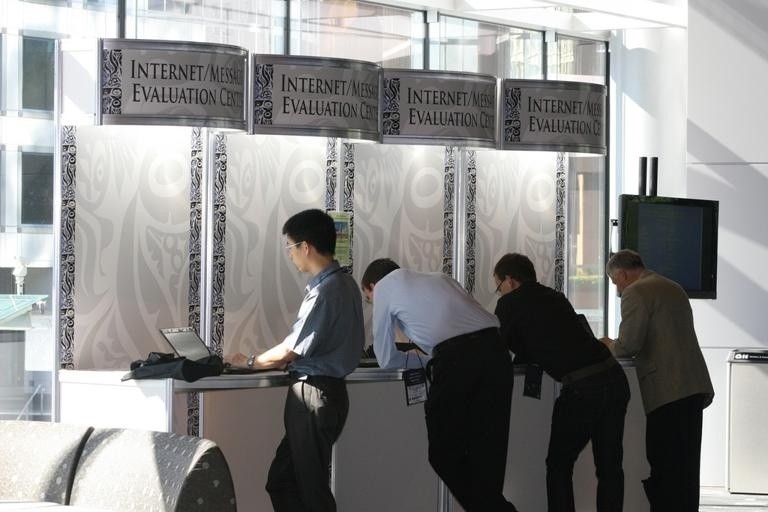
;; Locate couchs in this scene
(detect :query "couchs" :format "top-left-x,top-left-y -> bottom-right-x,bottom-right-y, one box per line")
0,419 -> 237,512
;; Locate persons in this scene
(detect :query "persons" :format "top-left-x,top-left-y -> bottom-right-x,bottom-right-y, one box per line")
361,255 -> 520,511
492,252 -> 632,511
597,246 -> 716,510
220,207 -> 367,511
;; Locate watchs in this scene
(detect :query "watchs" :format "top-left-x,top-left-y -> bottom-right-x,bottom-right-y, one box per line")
245,353 -> 257,371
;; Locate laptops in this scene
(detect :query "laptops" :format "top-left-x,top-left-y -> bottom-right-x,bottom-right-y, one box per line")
159,326 -> 258,374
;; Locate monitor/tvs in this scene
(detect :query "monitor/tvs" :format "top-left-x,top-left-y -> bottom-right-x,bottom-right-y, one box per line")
6,28 -> 68,120
0,260 -> 52,326
6,145 -> 53,235
616,194 -> 719,300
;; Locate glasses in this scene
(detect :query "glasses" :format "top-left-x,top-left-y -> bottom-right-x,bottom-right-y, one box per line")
494,279 -> 507,297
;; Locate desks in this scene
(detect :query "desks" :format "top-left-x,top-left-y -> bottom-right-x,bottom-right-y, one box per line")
55,348 -> 648,512
